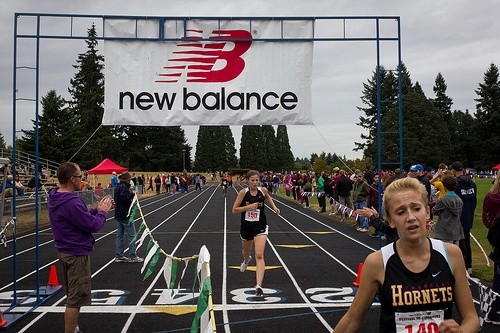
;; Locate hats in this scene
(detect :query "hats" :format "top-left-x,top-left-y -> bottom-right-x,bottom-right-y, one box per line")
450,162 -> 463,171
113,172 -> 116,175
413,164 -> 424,171
7,175 -> 13,179
426,167 -> 433,171
439,163 -> 445,170
410,165 -> 416,170
333,167 -> 340,171
395,168 -> 404,173
118,172 -> 134,181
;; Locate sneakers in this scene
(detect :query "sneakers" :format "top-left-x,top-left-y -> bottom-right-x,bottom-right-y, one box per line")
370,232 -> 379,237
129,256 -> 144,262
115,256 -> 129,262
357,228 -> 363,231
240,256 -> 252,272
256,286 -> 263,296
484,313 -> 500,325
360,228 -> 369,231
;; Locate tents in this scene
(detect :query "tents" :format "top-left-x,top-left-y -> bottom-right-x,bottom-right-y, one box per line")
85,158 -> 128,191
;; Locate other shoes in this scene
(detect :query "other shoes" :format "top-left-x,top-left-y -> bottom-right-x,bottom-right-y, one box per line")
303,206 -> 310,209
356,226 -> 359,230
316,208 -> 322,212
467,268 -> 472,274
329,212 -> 335,215
77,330 -> 83,333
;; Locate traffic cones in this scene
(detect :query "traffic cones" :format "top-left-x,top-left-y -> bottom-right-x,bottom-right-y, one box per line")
353,263 -> 364,286
0,311 -> 7,326
48,265 -> 58,286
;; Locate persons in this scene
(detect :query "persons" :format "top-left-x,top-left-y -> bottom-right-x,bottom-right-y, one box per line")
260,162 -> 500,282
332,178 -> 479,333
131,169 -> 234,198
232,170 -> 280,297
113,172 -> 144,262
47,162 -> 116,333
80,172 -> 118,199
0,156 -> 55,199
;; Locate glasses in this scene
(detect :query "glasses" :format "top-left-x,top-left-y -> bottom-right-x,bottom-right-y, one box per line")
68,175 -> 82,178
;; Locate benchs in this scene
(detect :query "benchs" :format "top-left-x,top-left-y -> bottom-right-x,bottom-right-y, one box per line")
0,165 -> 61,217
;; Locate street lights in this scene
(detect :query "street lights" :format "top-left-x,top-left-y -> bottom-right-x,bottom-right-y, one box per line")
182,149 -> 186,169
